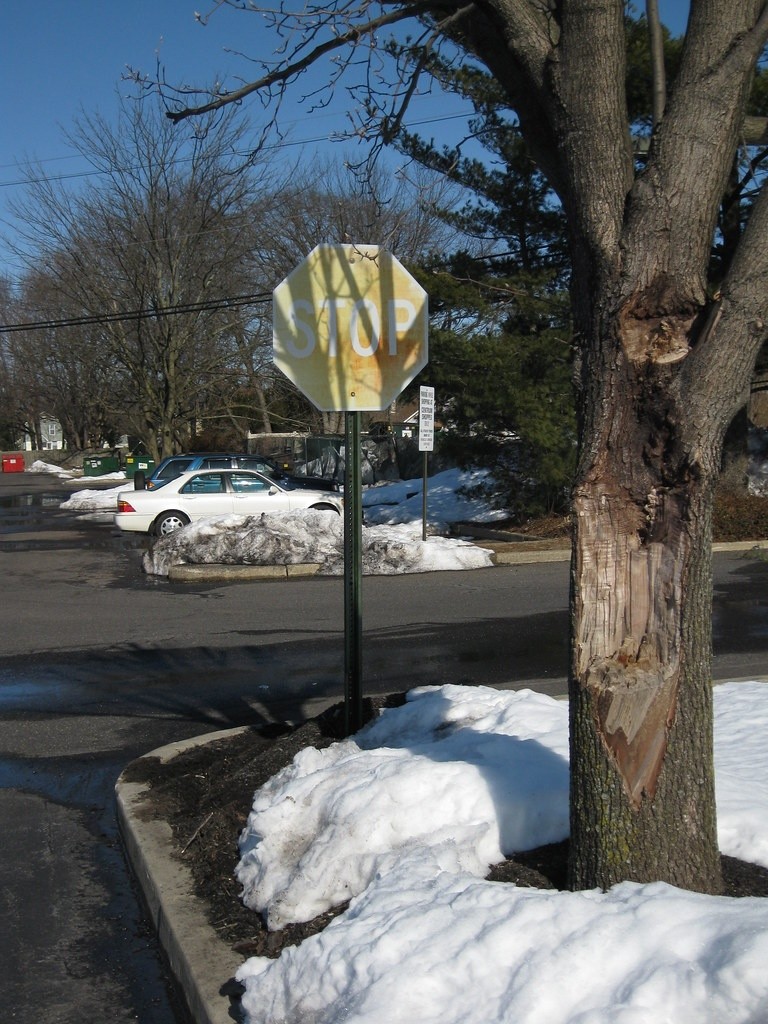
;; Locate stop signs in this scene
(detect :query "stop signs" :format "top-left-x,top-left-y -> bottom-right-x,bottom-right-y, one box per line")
271,242 -> 430,414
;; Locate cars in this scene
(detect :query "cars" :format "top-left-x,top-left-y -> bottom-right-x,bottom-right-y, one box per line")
111,468 -> 345,538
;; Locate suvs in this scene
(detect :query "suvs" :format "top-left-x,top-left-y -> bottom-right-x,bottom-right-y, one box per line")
134,451 -> 340,494
